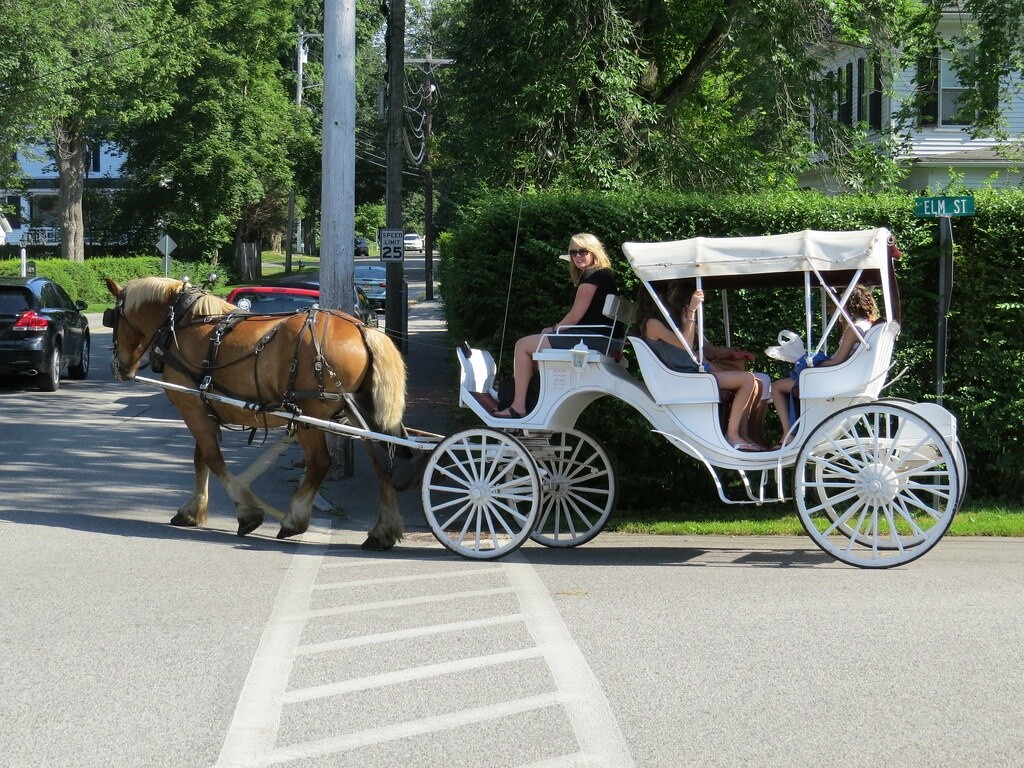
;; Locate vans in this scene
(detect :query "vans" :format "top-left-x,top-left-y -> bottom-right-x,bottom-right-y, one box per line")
354,237 -> 370,257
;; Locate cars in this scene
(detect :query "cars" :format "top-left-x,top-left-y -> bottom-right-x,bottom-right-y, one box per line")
225,286 -> 323,320
256,270 -> 381,330
342,265 -> 390,311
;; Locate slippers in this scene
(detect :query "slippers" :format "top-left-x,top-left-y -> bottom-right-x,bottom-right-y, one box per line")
731,440 -> 762,452
492,403 -> 528,418
769,441 -> 787,451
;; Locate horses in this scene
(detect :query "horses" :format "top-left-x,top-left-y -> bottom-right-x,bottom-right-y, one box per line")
104,276 -> 409,551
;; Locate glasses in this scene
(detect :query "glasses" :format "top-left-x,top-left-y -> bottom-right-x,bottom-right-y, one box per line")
570,249 -> 590,258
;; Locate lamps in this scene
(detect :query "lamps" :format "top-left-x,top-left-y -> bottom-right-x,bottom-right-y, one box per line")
569,337 -> 590,371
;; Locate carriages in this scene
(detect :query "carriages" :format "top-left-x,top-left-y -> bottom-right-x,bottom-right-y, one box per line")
98,226 -> 970,581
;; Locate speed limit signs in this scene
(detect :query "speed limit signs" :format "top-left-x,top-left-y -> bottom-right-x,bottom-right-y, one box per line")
380,229 -> 405,262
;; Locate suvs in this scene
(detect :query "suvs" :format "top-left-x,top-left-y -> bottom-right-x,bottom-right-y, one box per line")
0,274 -> 92,392
403,234 -> 423,254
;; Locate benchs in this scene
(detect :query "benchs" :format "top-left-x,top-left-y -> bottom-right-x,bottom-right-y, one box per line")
625,335 -> 733,408
531,293 -> 639,367
798,321 -> 901,400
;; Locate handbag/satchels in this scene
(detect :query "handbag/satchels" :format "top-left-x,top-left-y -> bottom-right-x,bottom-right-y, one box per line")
763,330 -> 806,363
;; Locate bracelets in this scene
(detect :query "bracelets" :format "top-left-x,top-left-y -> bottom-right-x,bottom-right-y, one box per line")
687,319 -> 696,322
552,324 -> 556,333
688,309 -> 695,312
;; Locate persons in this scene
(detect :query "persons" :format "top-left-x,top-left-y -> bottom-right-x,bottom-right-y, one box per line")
640,280 -> 771,452
495,233 -> 625,418
771,284 -> 881,450
